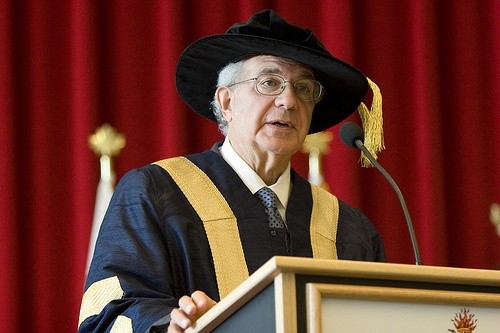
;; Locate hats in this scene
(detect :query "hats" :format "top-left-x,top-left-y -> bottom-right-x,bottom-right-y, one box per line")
175,9 -> 368,135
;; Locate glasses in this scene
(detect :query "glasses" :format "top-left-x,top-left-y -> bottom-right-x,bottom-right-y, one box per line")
223,74 -> 325,106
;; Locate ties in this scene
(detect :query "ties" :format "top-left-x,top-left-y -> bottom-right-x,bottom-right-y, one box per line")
256,186 -> 290,254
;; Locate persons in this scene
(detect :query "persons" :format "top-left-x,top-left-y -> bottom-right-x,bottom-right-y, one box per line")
78,7 -> 387,333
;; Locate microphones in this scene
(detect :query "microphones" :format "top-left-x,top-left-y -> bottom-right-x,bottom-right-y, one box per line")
339,121 -> 422,266
